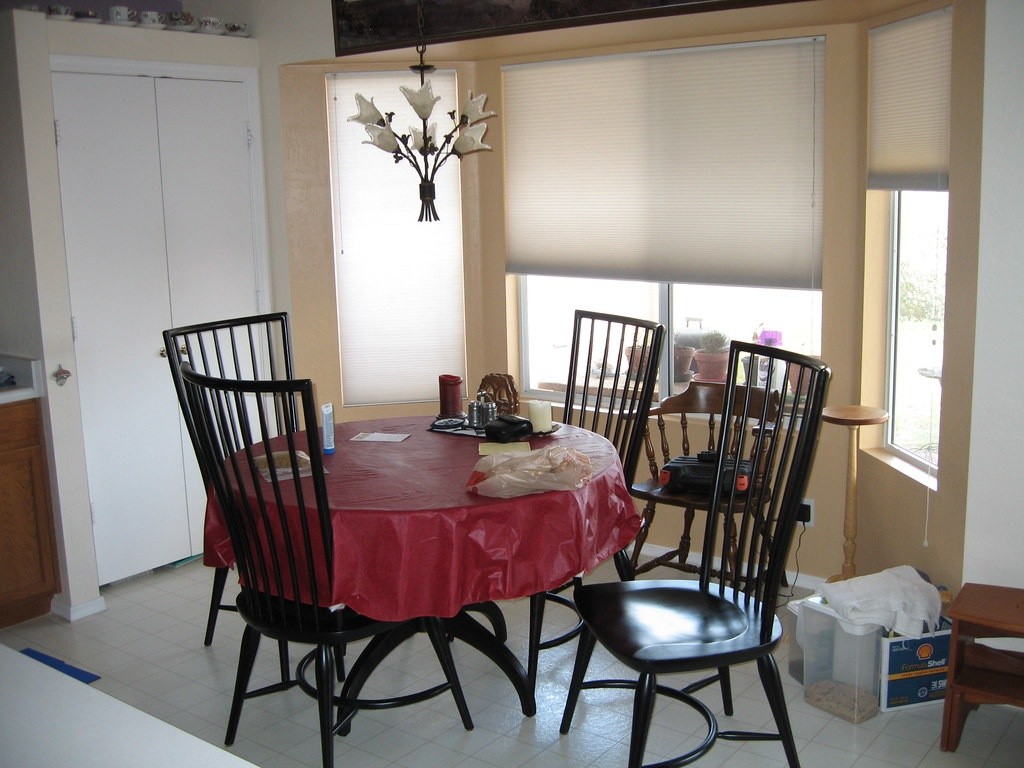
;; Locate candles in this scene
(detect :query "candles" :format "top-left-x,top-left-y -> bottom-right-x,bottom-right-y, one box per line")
527,400 -> 552,432
439,375 -> 462,415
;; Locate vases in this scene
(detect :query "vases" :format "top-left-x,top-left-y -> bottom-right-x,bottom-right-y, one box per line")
740,355 -> 760,387
787,355 -> 820,396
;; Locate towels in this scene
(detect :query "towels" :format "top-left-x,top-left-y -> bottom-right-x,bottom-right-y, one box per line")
821,563 -> 944,640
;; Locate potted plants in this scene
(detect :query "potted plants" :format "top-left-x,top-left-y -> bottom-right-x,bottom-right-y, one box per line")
625,331 -> 731,383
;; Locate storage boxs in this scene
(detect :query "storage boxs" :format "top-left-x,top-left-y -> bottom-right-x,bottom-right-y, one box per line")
787,594 -> 954,725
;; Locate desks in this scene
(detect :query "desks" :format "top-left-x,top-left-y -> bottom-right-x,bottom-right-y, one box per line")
0,644 -> 256,767
205,415 -> 643,738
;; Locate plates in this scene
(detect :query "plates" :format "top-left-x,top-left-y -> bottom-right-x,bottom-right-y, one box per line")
136,24 -> 167,30
197,29 -> 226,35
224,32 -> 251,38
75,19 -> 104,24
166,26 -> 197,32
106,21 -> 139,27
47,15 -> 75,21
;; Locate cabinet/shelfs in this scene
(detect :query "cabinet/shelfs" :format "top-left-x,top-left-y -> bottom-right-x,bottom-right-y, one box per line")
48,54 -> 280,587
0,397 -> 61,629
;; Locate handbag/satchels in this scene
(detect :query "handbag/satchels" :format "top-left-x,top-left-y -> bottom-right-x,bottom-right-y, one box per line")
464,446 -> 594,498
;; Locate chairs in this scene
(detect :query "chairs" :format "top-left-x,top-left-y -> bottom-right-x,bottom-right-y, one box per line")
940,582 -> 1024,752
528,309 -> 663,697
164,314 -> 346,684
621,382 -> 789,593
559,339 -> 833,768
477,372 -> 520,417
178,361 -> 473,768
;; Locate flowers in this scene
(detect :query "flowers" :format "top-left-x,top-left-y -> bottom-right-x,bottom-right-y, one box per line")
759,331 -> 783,347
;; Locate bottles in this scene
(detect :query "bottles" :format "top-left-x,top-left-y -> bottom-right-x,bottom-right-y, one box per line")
468,401 -> 482,426
482,402 -> 497,425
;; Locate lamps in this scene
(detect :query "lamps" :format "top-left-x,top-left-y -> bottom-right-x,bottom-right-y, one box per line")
346,0 -> 497,223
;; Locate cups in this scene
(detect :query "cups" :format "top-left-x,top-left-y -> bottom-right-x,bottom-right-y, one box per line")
140,11 -> 166,24
167,11 -> 194,26
49,5 -> 72,15
109,6 -> 137,21
225,22 -> 247,32
74,10 -> 103,18
439,375 -> 465,418
197,16 -> 224,29
20,4 -> 41,12
529,399 -> 552,433
